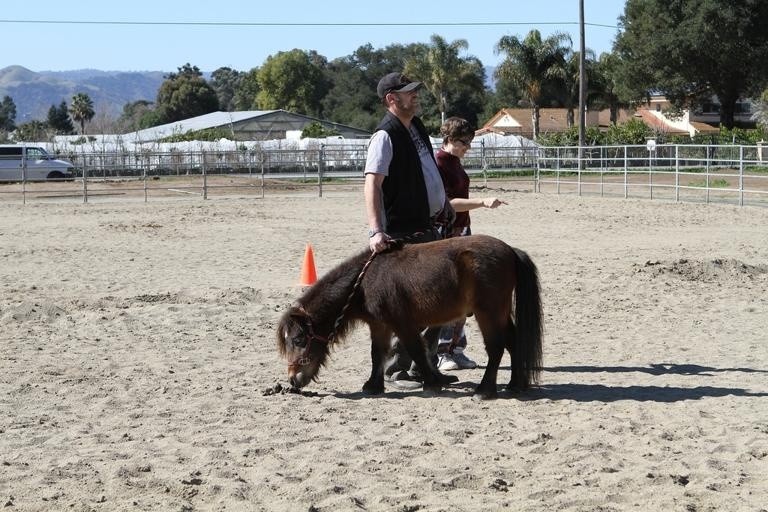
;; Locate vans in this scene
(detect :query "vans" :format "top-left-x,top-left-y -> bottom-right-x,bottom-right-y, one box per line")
0,143 -> 74,182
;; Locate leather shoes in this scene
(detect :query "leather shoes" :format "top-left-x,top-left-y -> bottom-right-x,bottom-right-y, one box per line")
386,379 -> 422,390
411,372 -> 460,383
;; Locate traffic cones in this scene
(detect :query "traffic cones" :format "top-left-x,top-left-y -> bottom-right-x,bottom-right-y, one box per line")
301,244 -> 319,286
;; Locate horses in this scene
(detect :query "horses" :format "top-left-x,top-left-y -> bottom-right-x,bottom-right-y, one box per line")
278,233 -> 545,401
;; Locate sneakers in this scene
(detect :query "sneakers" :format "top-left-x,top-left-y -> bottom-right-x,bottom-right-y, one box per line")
438,352 -> 477,370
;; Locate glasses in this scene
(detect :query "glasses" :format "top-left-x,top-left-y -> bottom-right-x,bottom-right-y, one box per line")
457,138 -> 470,146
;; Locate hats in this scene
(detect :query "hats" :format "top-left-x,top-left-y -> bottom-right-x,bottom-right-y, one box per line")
378,72 -> 422,100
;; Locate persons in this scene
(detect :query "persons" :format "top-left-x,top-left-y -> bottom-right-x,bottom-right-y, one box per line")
364,71 -> 462,391
433,116 -> 510,371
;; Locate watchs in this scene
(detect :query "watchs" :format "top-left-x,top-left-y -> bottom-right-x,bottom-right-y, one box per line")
368,226 -> 385,239
